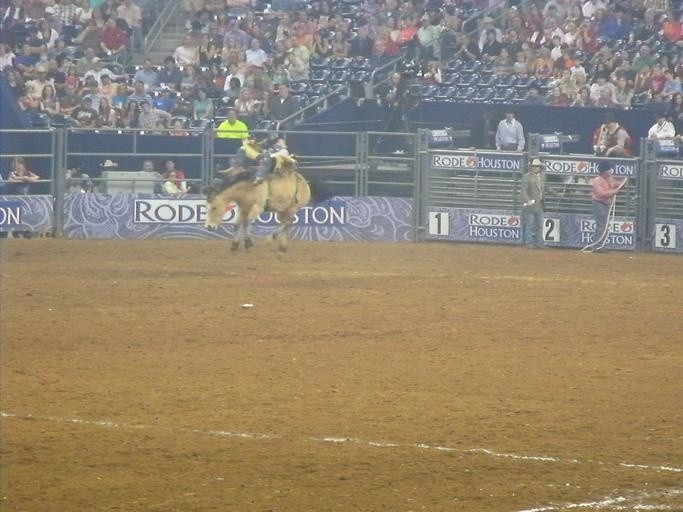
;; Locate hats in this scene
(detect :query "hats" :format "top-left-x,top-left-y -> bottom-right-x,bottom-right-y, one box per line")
610,4 -> 628,14
527,158 -> 546,166
503,104 -> 518,114
597,162 -> 612,171
600,113 -> 621,123
651,110 -> 673,120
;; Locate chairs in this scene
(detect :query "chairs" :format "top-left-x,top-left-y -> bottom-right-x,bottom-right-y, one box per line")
2,3 -> 683,131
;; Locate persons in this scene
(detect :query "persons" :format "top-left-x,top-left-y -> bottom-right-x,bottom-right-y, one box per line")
520,159 -> 560,250
591,162 -> 623,253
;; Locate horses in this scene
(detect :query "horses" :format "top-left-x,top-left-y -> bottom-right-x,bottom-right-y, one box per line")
200,166 -> 310,252
